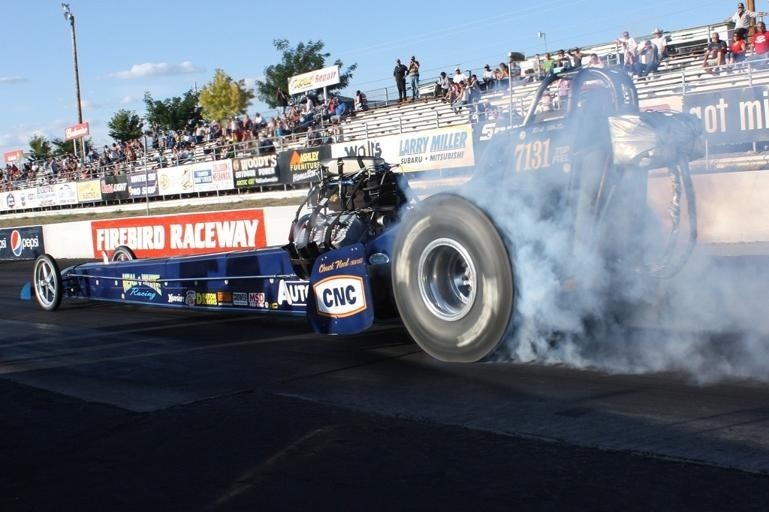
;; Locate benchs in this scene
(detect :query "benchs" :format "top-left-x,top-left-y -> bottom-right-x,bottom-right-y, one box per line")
135,44 -> 769,168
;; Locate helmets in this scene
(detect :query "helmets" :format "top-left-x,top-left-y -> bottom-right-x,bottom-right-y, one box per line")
559,79 -> 571,96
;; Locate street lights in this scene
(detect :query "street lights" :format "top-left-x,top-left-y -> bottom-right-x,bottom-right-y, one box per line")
144,129 -> 153,217
506,50 -> 526,130
538,31 -> 547,54
60,2 -> 84,125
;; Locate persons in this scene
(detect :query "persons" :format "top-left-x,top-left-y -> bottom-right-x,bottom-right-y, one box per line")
407,56 -> 421,101
703,32 -> 728,75
727,3 -> 768,50
751,21 -> 769,70
435,28 -> 668,123
725,32 -> 747,65
393,59 -> 409,102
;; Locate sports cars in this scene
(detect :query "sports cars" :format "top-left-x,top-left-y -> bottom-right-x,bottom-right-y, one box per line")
18,66 -> 709,366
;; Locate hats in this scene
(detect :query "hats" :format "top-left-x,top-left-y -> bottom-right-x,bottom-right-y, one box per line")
652,27 -> 663,34
